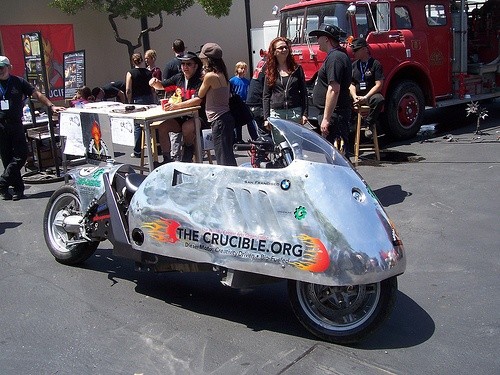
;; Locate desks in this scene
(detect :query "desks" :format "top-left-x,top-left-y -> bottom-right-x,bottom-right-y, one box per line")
22,113 -> 56,127
58,100 -> 203,183
26,124 -> 58,172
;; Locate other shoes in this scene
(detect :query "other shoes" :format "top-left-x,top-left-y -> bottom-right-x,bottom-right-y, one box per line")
0,192 -> 12,200
364,126 -> 373,138
131,153 -> 136,158
153,159 -> 168,168
13,194 -> 23,200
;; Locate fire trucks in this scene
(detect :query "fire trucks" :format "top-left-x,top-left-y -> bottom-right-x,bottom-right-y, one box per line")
274,0 -> 499,143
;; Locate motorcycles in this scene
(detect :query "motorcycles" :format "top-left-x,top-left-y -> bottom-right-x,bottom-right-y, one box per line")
43,117 -> 408,346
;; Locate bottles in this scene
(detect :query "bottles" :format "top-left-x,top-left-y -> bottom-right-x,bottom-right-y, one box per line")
24,105 -> 32,122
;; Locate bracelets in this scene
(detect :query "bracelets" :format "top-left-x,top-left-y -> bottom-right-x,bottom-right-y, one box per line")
49,103 -> 55,108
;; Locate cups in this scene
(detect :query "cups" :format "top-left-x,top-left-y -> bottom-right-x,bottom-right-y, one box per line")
160,99 -> 169,110
75,101 -> 85,109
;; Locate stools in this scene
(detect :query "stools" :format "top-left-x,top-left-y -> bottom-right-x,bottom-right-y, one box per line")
353,104 -> 380,163
138,121 -> 164,175
192,129 -> 215,163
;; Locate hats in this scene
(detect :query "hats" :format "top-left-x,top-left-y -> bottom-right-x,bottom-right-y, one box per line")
352,39 -> 368,50
176,52 -> 202,64
309,23 -> 341,42
92,87 -> 102,99
0,56 -> 10,67
199,42 -> 223,59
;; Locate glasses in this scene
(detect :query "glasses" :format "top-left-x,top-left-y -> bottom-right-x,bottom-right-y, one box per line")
276,46 -> 289,49
180,62 -> 192,66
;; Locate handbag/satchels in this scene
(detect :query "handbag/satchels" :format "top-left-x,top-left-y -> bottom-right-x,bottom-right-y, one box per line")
228,94 -> 254,127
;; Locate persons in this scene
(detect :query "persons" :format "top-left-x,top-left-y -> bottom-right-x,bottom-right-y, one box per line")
348,39 -> 385,137
263,36 -> 308,146
0,55 -> 66,201
244,37 -> 293,157
69,38 -> 237,166
228,60 -> 262,143
313,26 -> 352,166
